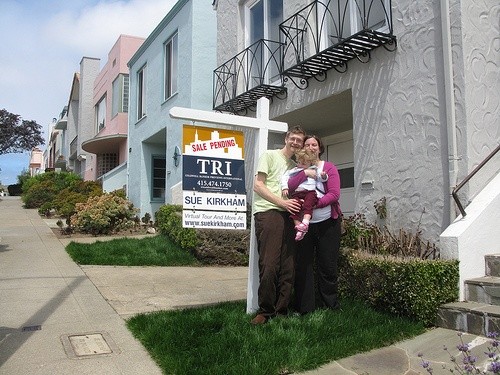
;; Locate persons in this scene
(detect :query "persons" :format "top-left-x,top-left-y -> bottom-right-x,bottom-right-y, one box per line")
281,145 -> 328,241
250,124 -> 307,325
291,132 -> 343,318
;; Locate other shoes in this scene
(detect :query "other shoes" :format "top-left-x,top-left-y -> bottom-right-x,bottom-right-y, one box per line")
249,315 -> 270,324
